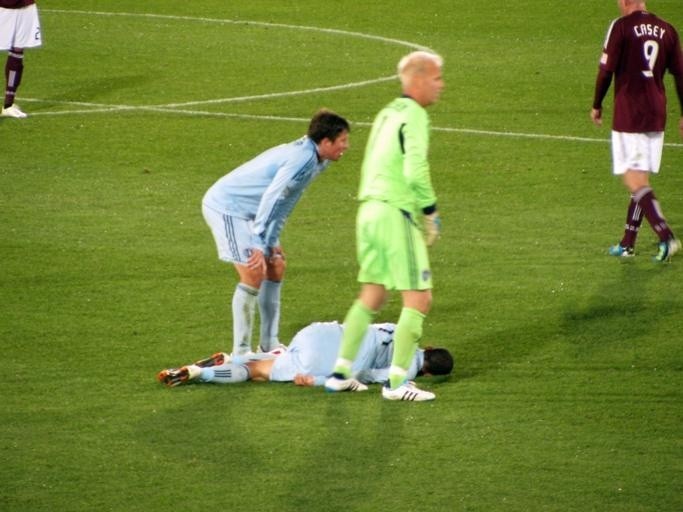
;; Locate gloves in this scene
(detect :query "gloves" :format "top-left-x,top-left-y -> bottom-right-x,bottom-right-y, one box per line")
423,204 -> 441,247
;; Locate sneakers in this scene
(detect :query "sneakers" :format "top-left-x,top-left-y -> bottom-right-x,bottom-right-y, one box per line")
608,242 -> 636,258
651,240 -> 682,264
156,364 -> 201,388
257,342 -> 290,357
231,348 -> 267,363
194,351 -> 230,367
324,376 -> 369,393
382,383 -> 436,402
1,105 -> 27,119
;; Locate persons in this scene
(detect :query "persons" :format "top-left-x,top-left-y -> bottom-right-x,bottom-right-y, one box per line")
588,1 -> 682,265
0,1 -> 43,120
323,50 -> 446,402
156,321 -> 453,387
199,107 -> 353,363
